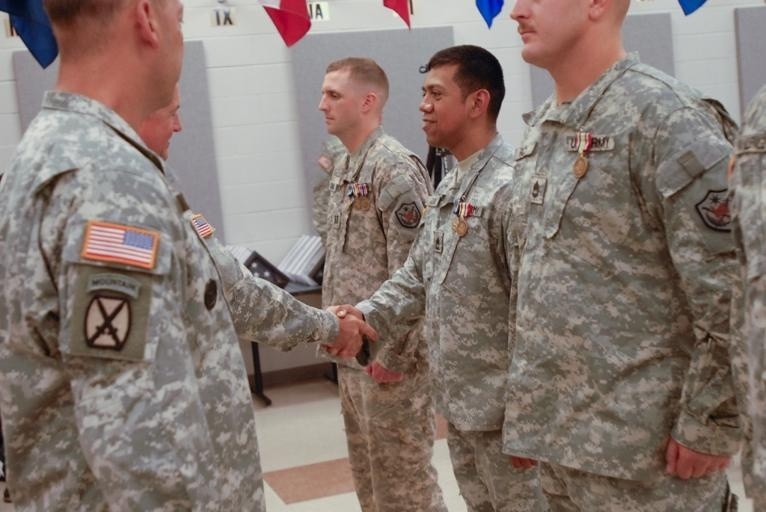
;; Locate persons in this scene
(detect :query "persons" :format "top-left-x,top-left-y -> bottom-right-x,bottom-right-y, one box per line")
322,44 -> 550,512
730,82 -> 765,510
0,0 -> 264,512
501,0 -> 745,512
314,57 -> 446,512
135,83 -> 377,359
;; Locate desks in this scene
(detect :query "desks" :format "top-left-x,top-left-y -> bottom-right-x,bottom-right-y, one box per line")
248,281 -> 338,405
